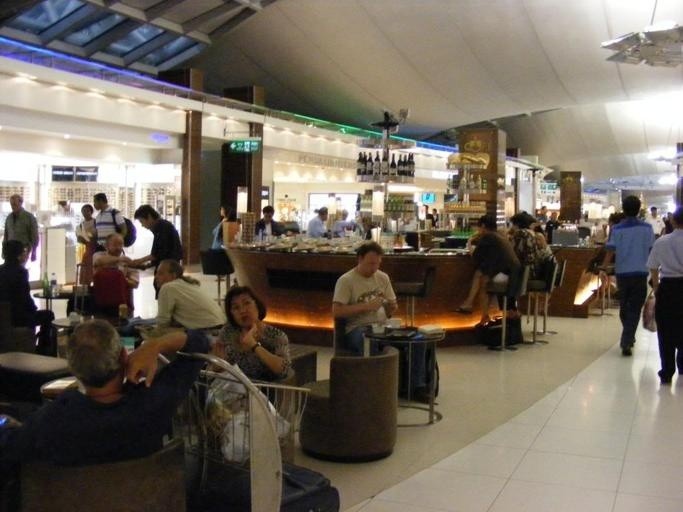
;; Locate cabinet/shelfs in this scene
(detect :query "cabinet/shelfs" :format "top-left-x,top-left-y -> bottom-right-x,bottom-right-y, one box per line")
356,138 -> 416,234
446,163 -> 487,248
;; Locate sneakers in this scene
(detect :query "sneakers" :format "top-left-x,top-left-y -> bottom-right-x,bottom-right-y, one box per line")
622,346 -> 632,356
661,377 -> 671,384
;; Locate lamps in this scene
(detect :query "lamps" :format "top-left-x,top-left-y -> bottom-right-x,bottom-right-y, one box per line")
601,0 -> 683,67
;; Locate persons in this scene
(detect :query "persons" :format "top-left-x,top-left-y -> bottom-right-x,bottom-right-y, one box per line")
211,203 -> 236,249
307,207 -> 355,239
586,195 -> 683,384
332,240 -> 398,355
534,205 -> 564,244
0,194 -> 227,358
0,319 -> 212,474
255,205 -> 282,236
211,283 -> 292,404
452,211 -> 559,328
423,204 -> 438,227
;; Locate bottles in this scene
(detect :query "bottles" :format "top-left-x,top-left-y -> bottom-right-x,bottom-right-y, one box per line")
42,271 -> 57,298
387,195 -> 415,211
590,229 -> 597,247
447,174 -> 487,236
358,150 -> 416,178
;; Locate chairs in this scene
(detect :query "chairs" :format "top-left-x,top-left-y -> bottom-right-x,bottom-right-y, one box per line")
486,258 -> 567,353
198,250 -> 235,305
0,302 -> 399,512
587,248 -> 621,316
393,264 -> 437,327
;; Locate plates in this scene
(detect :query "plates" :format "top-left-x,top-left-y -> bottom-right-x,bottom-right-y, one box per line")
393,246 -> 414,250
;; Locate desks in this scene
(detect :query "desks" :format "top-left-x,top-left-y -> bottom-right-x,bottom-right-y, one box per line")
365,328 -> 448,428
32,290 -> 129,334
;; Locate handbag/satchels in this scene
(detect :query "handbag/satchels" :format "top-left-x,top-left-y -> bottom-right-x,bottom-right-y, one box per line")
484,317 -> 523,345
543,254 -> 555,278
643,296 -> 657,332
111,209 -> 136,247
380,342 -> 439,404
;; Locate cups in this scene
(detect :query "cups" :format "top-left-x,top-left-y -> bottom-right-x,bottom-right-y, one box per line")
119,304 -> 128,318
389,318 -> 401,330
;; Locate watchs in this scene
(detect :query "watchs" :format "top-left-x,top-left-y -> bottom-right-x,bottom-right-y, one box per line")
252,342 -> 262,352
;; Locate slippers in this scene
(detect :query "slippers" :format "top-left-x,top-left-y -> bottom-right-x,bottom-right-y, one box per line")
452,307 -> 491,327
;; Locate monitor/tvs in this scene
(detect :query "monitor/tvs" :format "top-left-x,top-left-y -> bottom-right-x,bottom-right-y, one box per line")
421,192 -> 436,204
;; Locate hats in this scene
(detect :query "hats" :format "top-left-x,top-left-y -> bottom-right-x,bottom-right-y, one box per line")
511,211 -> 536,225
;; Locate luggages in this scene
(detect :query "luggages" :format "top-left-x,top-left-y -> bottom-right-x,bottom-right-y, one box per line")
185,462 -> 339,511
